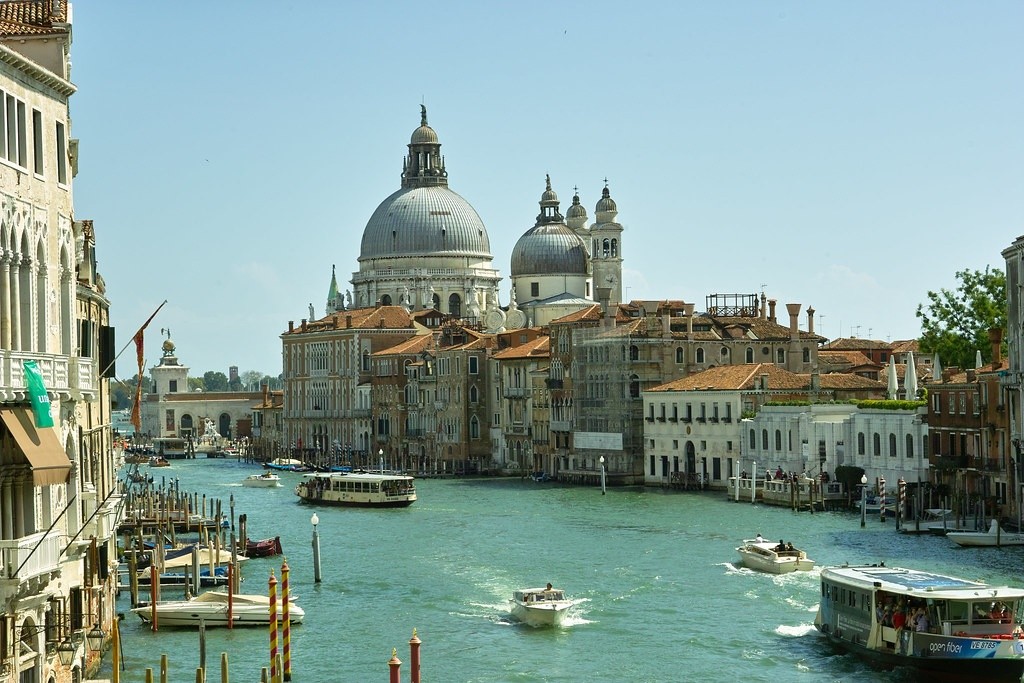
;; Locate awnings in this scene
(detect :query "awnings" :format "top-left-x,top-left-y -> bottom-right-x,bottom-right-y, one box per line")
0,406 -> 73,486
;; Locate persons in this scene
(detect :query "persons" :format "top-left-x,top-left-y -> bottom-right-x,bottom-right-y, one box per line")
776,540 -> 785,551
1018,624 -> 1024,639
992,602 -> 1002,619
755,534 -> 762,543
742,469 -> 747,479
820,472 -> 830,483
542,583 -> 553,600
776,466 -> 782,478
787,542 -> 793,551
766,470 -> 772,481
267,471 -> 271,477
877,600 -> 929,633
301,476 -> 332,493
788,471 -> 793,481
793,472 -> 799,482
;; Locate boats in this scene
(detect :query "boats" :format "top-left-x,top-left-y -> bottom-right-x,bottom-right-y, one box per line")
135,536 -> 282,583
946,518 -> 1024,548
125,469 -> 154,483
293,472 -> 418,506
508,588 -> 574,627
147,457 -> 171,467
240,473 -> 282,488
927,526 -> 980,537
734,538 -> 816,574
264,457 -> 361,473
856,496 -> 901,515
128,592 -> 306,627
812,561 -> 1024,683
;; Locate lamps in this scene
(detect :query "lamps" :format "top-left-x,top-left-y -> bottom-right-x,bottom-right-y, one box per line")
45,613 -> 105,650
13,625 -> 77,669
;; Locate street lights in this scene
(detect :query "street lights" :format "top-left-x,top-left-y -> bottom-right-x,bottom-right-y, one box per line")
378,448 -> 385,475
599,455 -> 606,496
861,474 -> 868,527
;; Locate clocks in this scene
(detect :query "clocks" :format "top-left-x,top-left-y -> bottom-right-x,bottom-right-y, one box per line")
605,274 -> 618,290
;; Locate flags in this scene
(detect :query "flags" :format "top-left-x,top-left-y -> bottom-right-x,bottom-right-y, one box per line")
24,361 -> 54,428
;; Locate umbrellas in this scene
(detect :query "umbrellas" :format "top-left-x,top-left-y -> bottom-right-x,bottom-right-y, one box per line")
887,355 -> 899,400
905,351 -> 918,400
976,351 -> 982,368
933,353 -> 942,381
